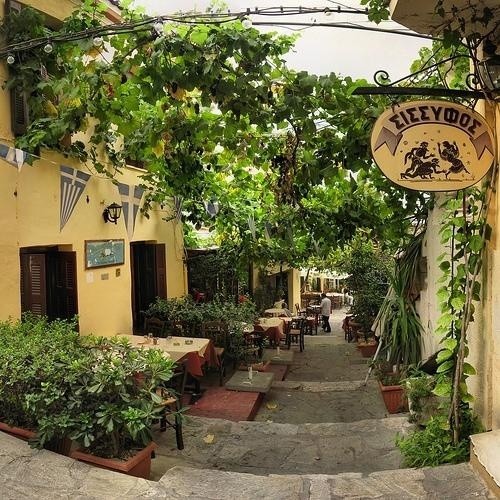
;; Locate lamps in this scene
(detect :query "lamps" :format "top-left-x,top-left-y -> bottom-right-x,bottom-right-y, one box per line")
475,36 -> 500,102
102,202 -> 123,225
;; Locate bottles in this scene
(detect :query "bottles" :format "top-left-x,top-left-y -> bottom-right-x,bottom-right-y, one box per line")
144,333 -> 172,345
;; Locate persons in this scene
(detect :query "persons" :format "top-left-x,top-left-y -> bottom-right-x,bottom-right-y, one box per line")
316,293 -> 332,333
281,303 -> 294,317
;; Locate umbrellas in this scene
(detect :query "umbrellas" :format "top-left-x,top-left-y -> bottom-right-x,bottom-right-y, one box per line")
362,238 -> 422,386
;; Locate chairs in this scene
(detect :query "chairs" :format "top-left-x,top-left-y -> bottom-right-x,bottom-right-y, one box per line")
262,287 -> 334,354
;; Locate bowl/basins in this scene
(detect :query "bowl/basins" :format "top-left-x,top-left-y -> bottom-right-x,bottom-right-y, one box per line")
184,339 -> 193,344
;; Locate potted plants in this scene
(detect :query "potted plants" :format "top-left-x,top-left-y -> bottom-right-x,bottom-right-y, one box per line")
342,228 -> 472,431
